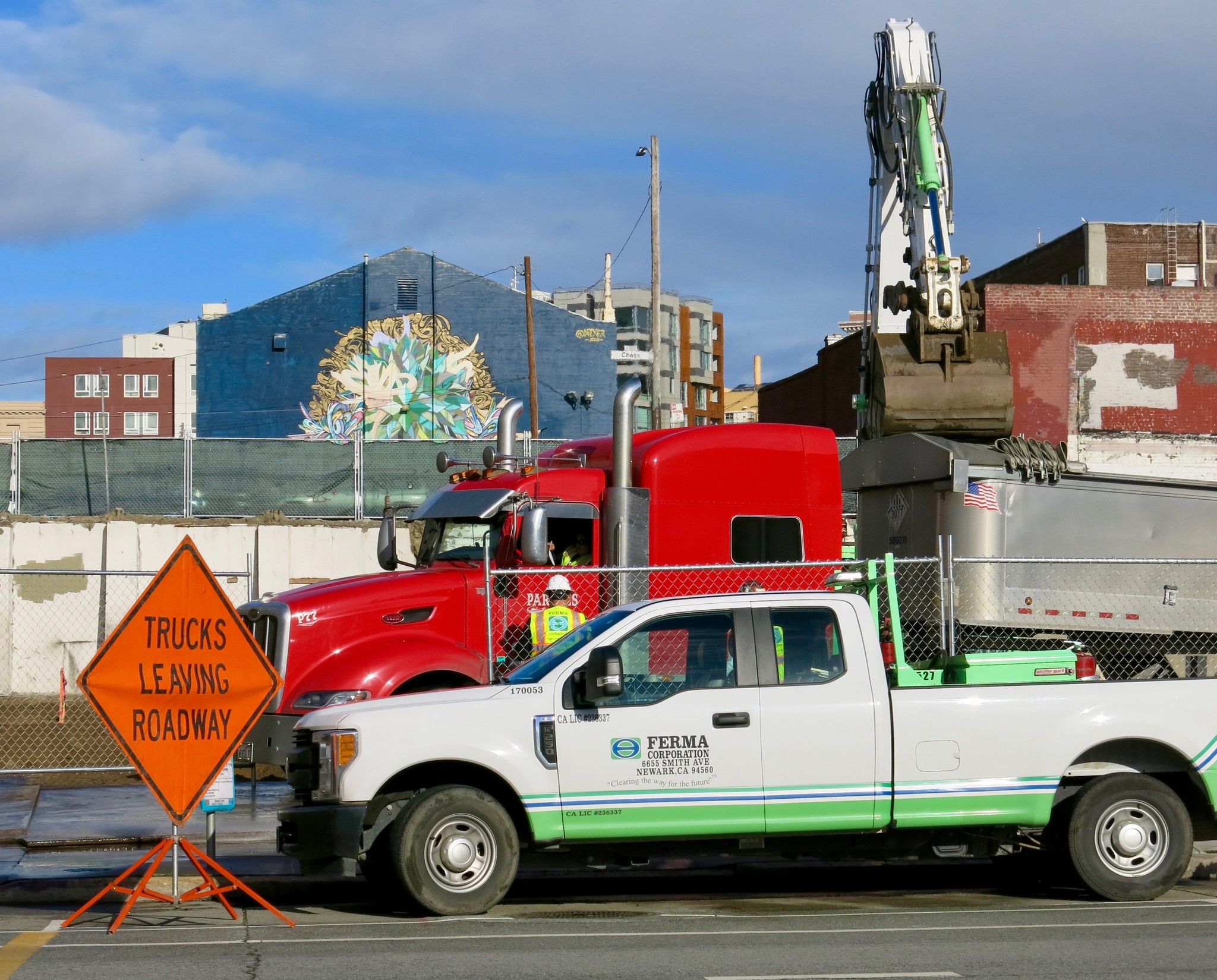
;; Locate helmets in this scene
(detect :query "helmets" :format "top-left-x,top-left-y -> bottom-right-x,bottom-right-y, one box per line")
544,575 -> 575,595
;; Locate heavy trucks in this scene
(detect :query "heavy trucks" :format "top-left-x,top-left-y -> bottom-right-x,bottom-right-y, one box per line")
234,376 -> 1215,764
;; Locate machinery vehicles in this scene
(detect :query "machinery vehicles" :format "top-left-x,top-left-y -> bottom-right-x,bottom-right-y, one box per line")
837,14 -> 1100,684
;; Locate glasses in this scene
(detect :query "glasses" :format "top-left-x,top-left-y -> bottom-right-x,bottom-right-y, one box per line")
576,537 -> 590,545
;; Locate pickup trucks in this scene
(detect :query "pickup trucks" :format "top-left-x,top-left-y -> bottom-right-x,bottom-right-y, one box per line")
277,552 -> 1217,917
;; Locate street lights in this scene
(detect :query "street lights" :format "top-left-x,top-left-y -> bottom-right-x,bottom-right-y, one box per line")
636,146 -> 661,429
521,271 -> 539,440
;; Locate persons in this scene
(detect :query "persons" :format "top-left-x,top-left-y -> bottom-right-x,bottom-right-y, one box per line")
732,581 -> 786,688
537,522 -> 593,570
503,576 -> 589,663
